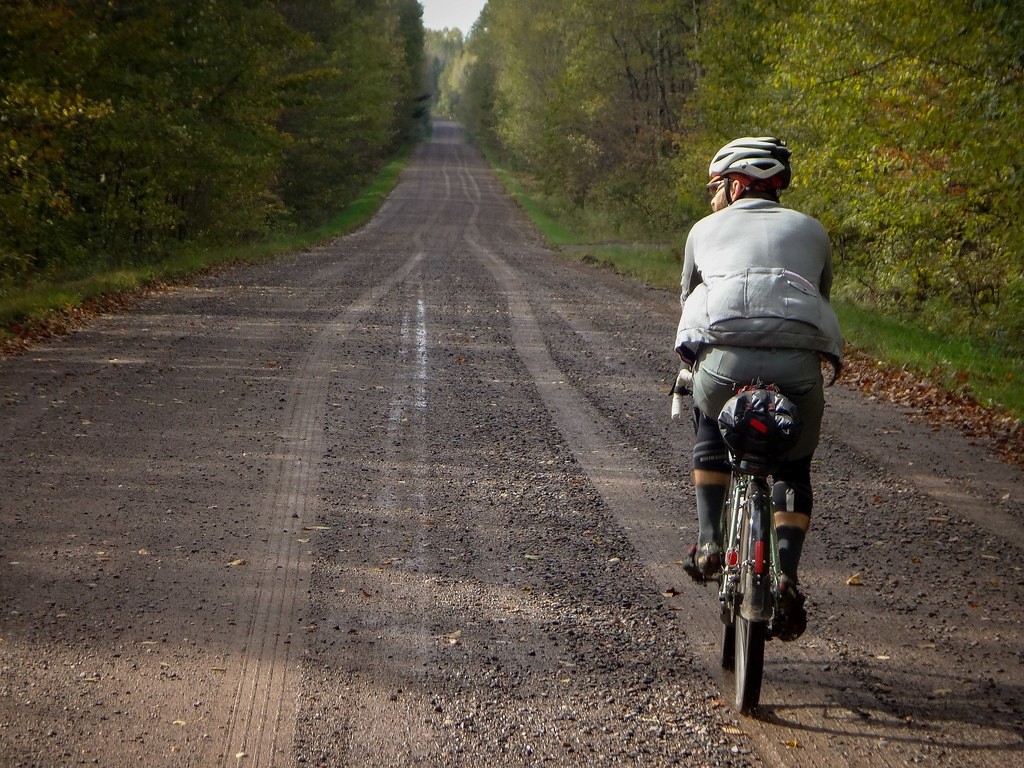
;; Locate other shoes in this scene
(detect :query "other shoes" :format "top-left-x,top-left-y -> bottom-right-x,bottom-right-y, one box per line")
694,542 -> 724,573
775,575 -> 806,641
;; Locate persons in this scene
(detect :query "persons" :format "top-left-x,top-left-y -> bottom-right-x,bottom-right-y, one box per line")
675,137 -> 843,640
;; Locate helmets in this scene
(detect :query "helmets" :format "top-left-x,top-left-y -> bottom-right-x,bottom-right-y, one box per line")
709,136 -> 792,189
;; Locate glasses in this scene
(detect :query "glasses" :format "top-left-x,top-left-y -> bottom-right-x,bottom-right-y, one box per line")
707,178 -> 749,195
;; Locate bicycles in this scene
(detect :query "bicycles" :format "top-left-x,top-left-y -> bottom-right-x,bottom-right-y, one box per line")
675,349 -> 806,716
